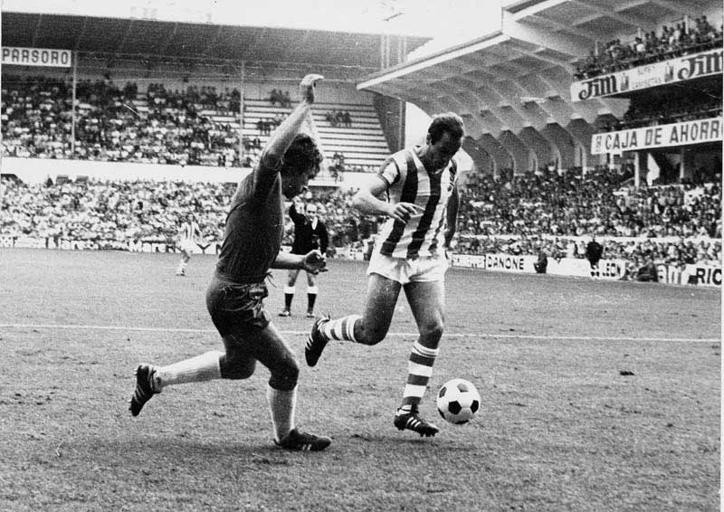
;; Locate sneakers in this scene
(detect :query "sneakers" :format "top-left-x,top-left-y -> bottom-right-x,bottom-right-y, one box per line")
303,313 -> 331,369
391,411 -> 441,438
272,425 -> 334,453
279,308 -> 317,319
127,361 -> 162,418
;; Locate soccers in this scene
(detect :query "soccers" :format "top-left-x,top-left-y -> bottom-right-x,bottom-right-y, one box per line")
437,378 -> 481,425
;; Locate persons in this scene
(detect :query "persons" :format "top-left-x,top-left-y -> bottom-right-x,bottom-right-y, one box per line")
305,114 -> 467,436
326,108 -> 353,129
1,176 -> 240,249
280,185 -> 390,258
453,158 -> 724,284
174,211 -> 202,276
1,69 -> 293,168
279,183 -> 336,319
572,15 -> 724,83
330,151 -> 377,183
593,86 -> 724,129
127,74 -> 330,451
